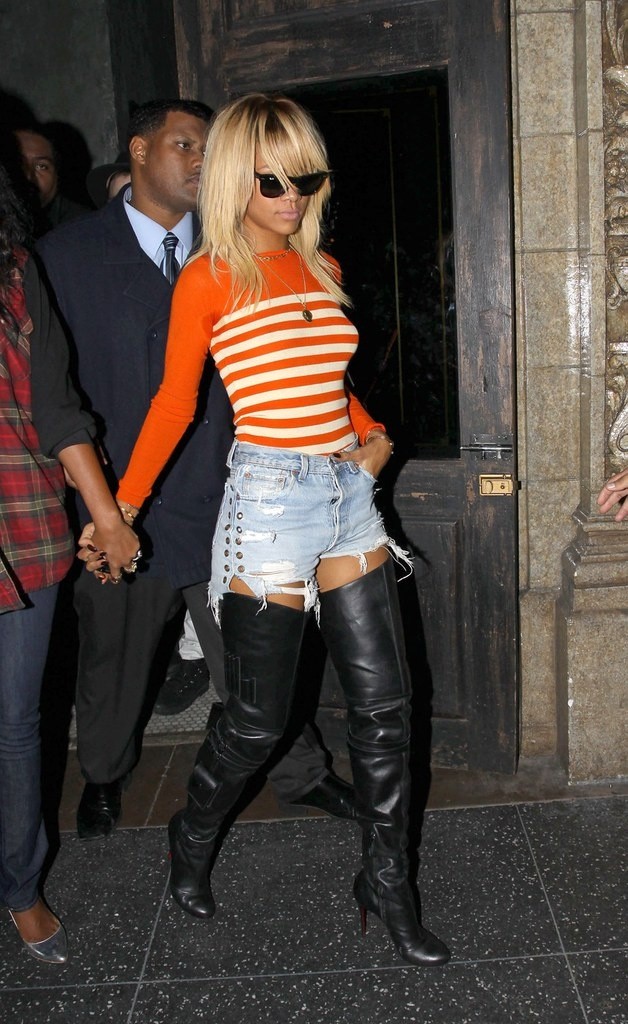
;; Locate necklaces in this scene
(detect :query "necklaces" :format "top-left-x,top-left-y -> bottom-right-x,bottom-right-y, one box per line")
254,244 -> 313,322
259,248 -> 291,261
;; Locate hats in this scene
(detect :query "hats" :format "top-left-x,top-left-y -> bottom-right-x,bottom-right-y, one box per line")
86,152 -> 134,209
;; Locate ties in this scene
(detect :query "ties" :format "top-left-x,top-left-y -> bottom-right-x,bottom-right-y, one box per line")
160,232 -> 180,285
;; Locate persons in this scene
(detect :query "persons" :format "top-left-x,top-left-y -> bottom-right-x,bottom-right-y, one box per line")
75,93 -> 452,967
0,100 -> 357,964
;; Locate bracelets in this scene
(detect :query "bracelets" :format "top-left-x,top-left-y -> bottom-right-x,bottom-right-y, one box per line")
367,436 -> 394,455
118,505 -> 135,520
128,503 -> 139,514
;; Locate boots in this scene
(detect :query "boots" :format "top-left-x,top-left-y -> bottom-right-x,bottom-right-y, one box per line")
314,554 -> 451,967
168,592 -> 310,918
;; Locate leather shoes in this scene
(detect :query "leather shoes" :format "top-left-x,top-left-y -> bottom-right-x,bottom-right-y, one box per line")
279,772 -> 359,823
76,771 -> 133,842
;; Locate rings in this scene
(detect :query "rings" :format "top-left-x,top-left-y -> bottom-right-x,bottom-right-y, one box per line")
124,562 -> 137,573
109,572 -> 122,584
132,550 -> 142,561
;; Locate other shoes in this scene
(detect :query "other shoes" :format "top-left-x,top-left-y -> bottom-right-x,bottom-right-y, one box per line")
155,658 -> 210,716
8,908 -> 69,964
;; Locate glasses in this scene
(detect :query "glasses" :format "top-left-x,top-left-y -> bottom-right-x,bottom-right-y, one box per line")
253,168 -> 328,198
